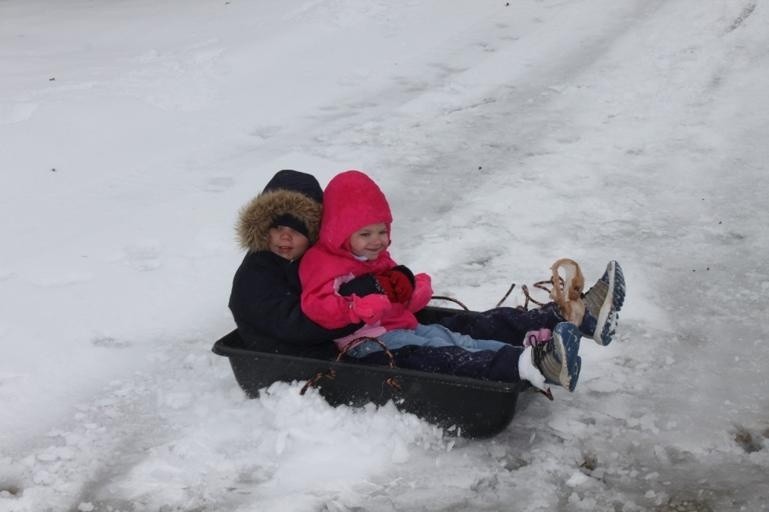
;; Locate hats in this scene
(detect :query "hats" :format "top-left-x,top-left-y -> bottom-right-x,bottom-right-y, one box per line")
269,213 -> 309,238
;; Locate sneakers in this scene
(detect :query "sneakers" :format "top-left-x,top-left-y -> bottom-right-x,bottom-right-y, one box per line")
578,261 -> 626,347
528,321 -> 581,393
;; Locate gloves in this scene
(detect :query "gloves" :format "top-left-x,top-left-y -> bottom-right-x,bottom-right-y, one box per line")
346,266 -> 433,324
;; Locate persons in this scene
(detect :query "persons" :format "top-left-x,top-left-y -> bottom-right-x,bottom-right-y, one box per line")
230,169 -> 627,400
298,170 -> 550,359
